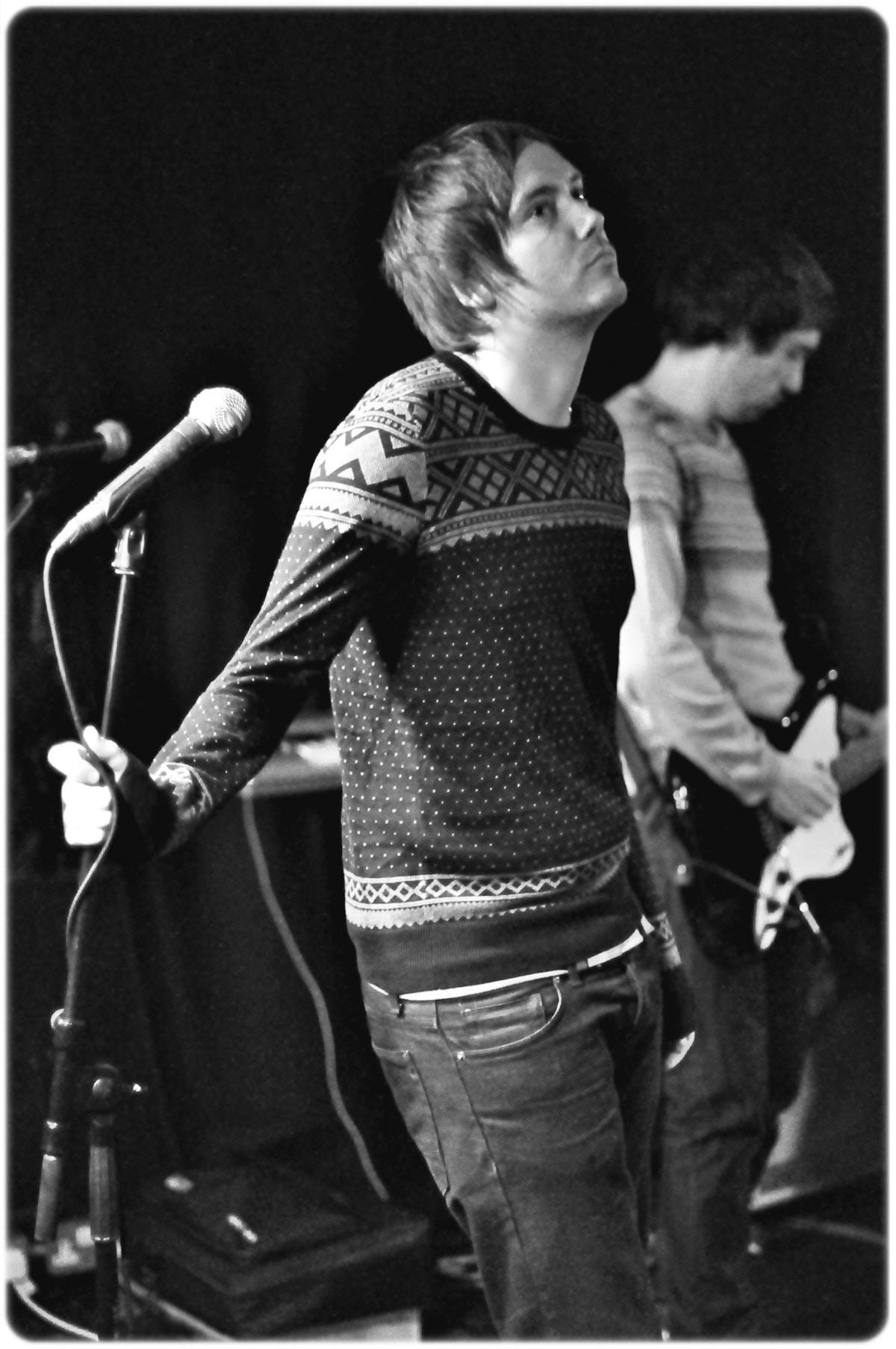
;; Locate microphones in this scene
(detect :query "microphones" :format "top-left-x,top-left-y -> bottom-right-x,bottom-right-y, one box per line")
51,387 -> 251,550
6,420 -> 133,468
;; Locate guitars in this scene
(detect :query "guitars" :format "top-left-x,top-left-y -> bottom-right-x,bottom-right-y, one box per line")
666,667 -> 889,983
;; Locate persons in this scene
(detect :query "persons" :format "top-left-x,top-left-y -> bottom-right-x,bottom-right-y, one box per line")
601,218 -> 887,1256
48,119 -> 778,1340
